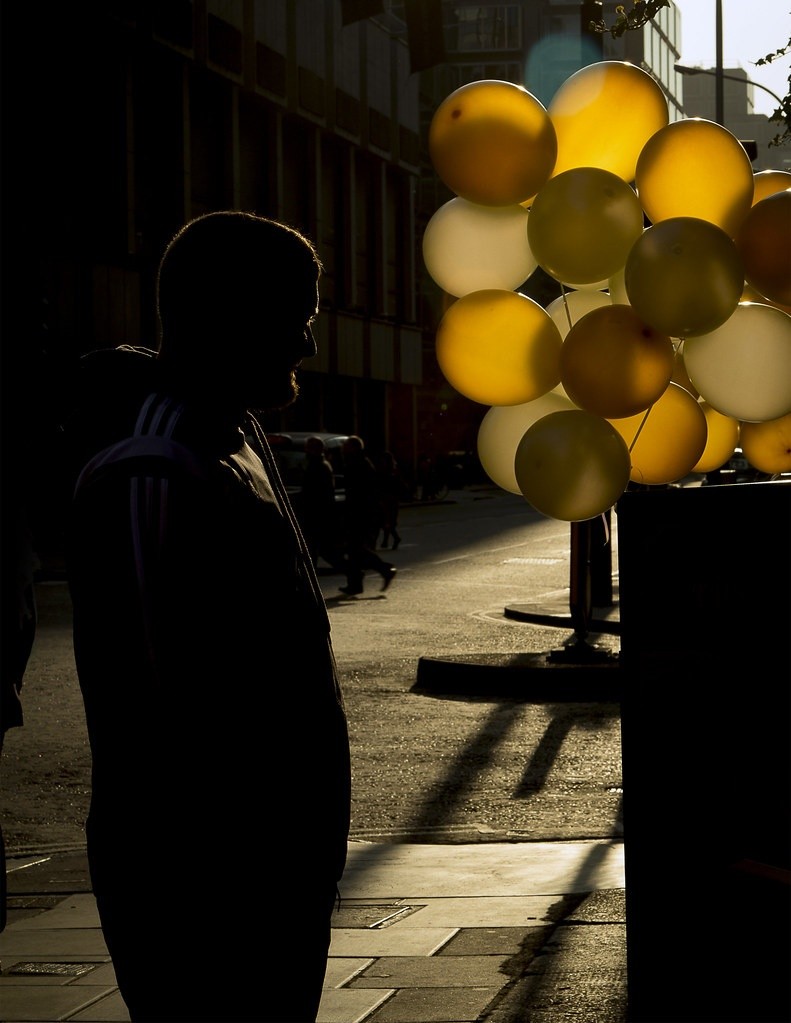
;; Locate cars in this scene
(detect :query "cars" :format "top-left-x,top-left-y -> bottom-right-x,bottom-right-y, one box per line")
261,432 -> 383,571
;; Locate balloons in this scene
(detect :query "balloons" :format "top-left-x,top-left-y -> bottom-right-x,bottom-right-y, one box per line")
422,58 -> 790,523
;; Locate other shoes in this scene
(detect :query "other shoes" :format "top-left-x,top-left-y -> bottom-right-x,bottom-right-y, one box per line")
380,568 -> 398,591
339,586 -> 363,594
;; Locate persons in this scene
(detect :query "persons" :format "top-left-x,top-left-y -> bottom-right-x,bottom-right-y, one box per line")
70,211 -> 351,1022
295,438 -> 400,595
